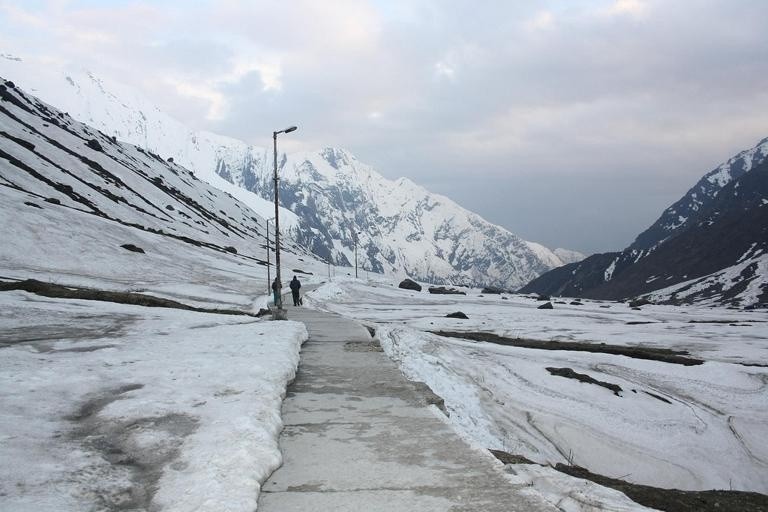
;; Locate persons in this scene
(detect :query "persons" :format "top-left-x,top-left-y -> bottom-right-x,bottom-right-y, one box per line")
270,276 -> 282,307
288,274 -> 302,306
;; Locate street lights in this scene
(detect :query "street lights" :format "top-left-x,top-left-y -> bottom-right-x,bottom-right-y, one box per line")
353,230 -> 363,279
266,125 -> 299,298
